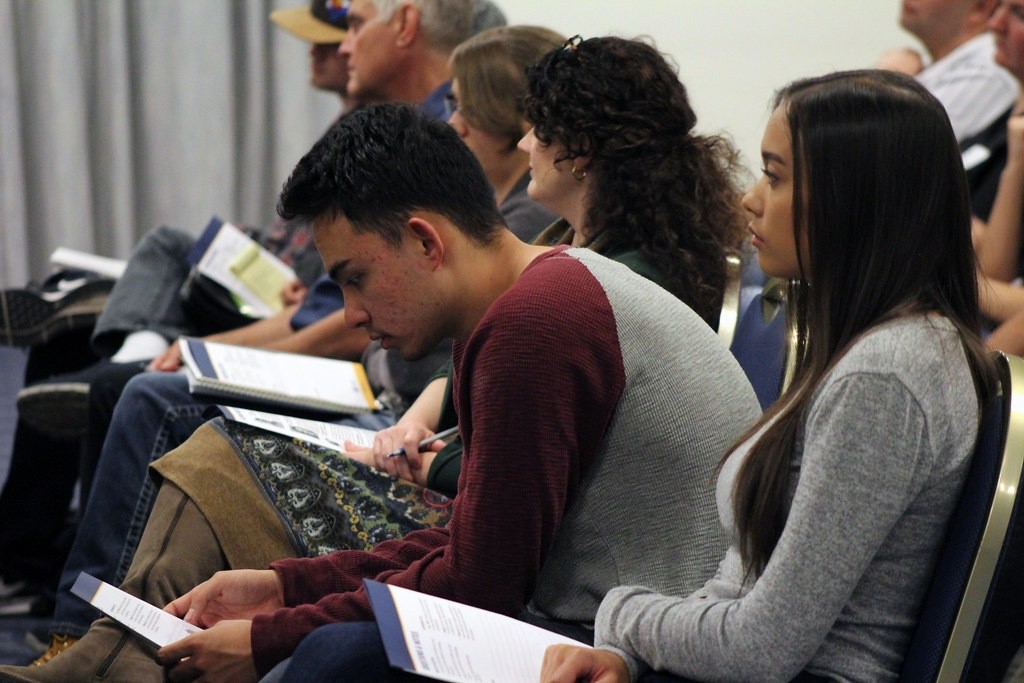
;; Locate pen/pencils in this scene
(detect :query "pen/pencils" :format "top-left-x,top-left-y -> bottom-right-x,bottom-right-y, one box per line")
387,424 -> 459,458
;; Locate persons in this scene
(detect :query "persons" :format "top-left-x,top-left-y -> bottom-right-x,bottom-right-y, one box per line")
0,0 -> 1024,683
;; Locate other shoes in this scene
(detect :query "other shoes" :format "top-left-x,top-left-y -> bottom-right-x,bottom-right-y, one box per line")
0,278 -> 118,350
28,631 -> 81,669
16,381 -> 93,425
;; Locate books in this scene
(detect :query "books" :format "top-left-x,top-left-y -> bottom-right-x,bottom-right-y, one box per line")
178,335 -> 384,415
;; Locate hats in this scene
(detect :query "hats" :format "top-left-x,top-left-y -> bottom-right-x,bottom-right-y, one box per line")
269,0 -> 353,44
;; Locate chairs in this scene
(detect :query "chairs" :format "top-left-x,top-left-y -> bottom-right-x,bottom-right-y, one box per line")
705,249 -> 1024,683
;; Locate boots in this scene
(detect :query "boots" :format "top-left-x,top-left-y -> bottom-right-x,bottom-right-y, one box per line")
0,474 -> 227,683
